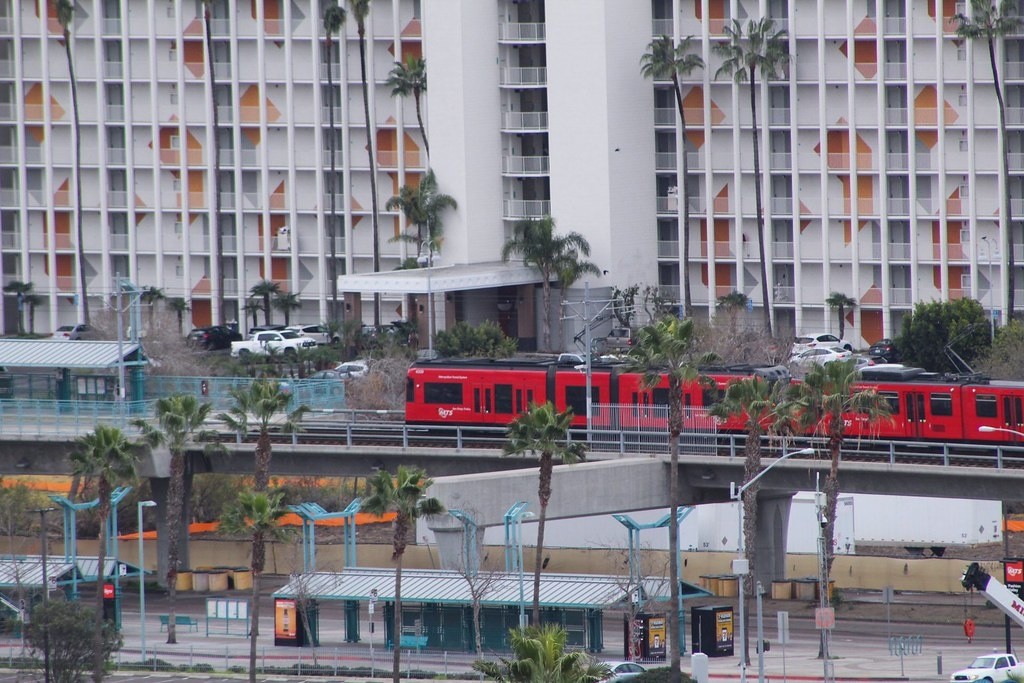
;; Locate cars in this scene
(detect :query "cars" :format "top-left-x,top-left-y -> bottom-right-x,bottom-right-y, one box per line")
786,346 -> 853,368
847,355 -> 888,370
557,351 -> 630,366
49,323 -> 108,341
790,334 -> 855,355
868,338 -> 896,359
414,349 -> 437,360
246,324 -> 400,345
592,661 -> 650,683
301,356 -> 374,382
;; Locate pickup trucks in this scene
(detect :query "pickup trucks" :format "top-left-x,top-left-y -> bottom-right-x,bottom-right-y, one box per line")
948,654 -> 1023,681
231,329 -> 318,358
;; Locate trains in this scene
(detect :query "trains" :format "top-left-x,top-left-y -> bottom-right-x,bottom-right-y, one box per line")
400,355 -> 1024,448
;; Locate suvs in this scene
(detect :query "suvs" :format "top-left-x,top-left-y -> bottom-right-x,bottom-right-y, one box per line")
185,326 -> 244,350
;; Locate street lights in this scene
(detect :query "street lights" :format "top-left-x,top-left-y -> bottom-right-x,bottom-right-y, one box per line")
138,500 -> 158,667
739,447 -> 815,683
417,237 -> 440,363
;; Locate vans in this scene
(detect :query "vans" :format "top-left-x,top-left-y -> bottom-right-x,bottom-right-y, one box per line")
603,324 -> 644,353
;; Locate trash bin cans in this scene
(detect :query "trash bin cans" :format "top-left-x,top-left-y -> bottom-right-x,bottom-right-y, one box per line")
698,574 -> 739,597
771,577 -> 836,601
175,564 -> 254,592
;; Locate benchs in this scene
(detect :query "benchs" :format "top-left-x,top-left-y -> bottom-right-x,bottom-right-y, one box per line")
388,635 -> 429,653
159,615 -> 199,633
